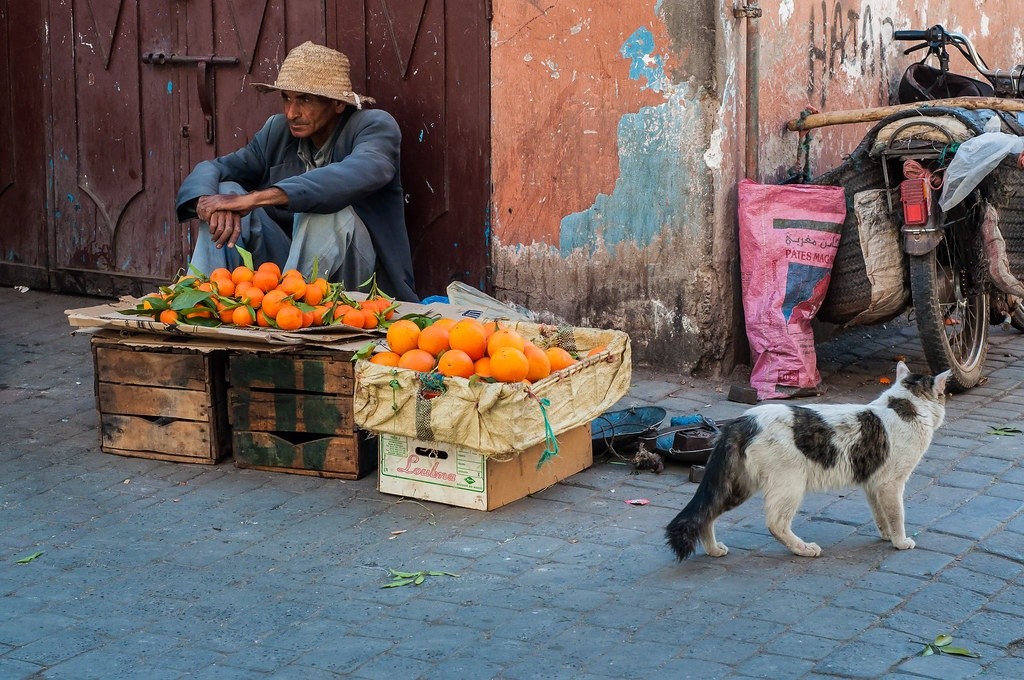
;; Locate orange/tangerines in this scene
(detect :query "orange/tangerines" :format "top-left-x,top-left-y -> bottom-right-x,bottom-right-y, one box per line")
370,320 -> 574,384
144,262 -> 394,329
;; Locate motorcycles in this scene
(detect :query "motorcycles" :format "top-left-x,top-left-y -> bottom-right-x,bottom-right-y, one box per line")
887,26 -> 1024,392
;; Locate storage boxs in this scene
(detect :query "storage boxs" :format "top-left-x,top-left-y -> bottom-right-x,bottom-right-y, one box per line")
90,320 -> 631,512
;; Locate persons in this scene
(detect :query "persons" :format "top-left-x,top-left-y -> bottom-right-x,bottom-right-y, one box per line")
175,41 -> 421,306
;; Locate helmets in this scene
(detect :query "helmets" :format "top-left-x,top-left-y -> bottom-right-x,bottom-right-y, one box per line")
898,61 -> 995,115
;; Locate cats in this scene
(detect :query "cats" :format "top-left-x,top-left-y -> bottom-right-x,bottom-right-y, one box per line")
662,358 -> 956,565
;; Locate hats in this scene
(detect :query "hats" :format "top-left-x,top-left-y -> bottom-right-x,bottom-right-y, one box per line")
248,40 -> 377,110
